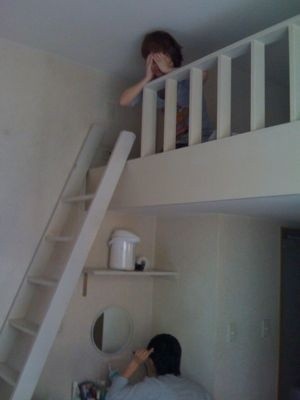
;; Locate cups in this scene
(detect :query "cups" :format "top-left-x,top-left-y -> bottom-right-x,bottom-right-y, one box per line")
107,229 -> 140,271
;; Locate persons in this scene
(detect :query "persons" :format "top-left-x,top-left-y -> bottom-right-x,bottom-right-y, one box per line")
120,31 -> 212,153
105,333 -> 214,400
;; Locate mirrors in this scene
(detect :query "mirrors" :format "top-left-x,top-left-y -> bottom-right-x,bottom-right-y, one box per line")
90,305 -> 134,358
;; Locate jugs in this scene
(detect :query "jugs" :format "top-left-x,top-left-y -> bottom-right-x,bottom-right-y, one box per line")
78,380 -> 106,400
